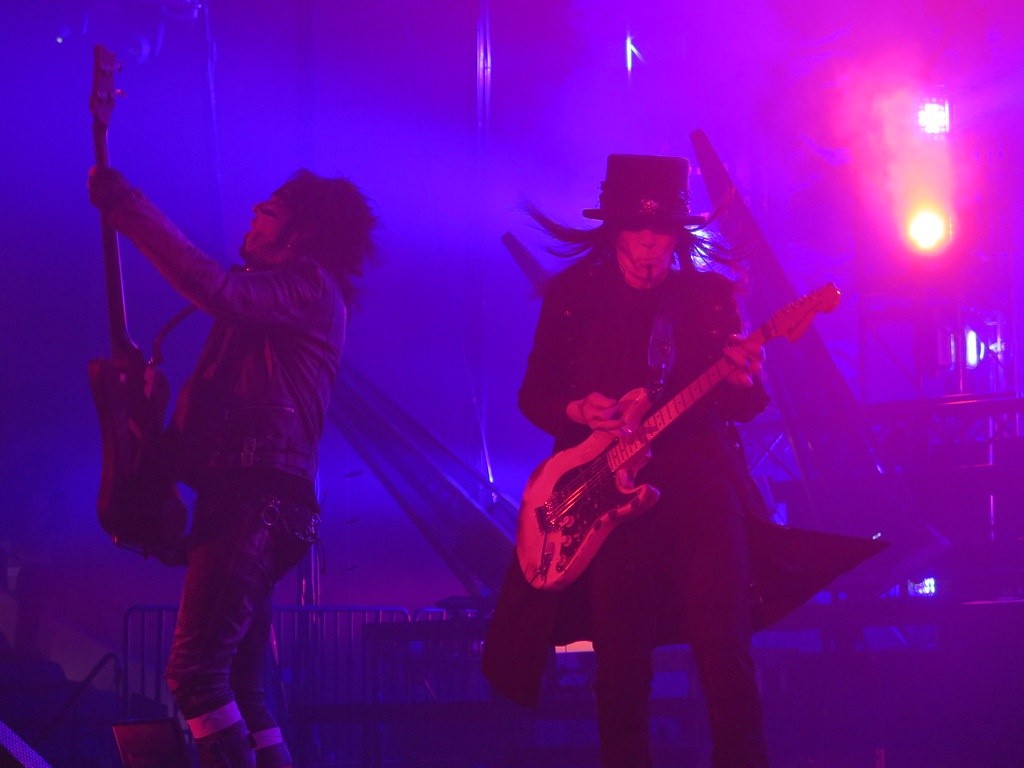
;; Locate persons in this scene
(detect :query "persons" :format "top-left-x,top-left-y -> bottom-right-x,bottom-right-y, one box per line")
482,154 -> 893,768
89,165 -> 379,768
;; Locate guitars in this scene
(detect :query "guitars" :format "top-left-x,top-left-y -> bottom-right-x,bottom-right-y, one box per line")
514,280 -> 845,594
84,47 -> 188,551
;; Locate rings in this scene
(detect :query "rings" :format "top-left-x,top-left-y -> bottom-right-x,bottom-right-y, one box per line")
755,349 -> 767,360
737,354 -> 753,373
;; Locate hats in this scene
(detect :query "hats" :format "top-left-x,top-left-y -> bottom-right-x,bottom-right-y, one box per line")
581,154 -> 710,227
